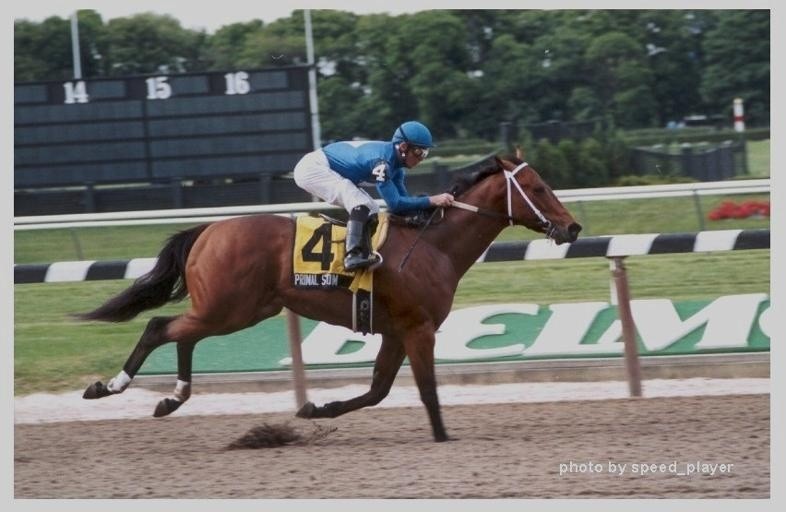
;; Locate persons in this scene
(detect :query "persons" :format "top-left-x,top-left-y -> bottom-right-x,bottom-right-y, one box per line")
293,121 -> 454,272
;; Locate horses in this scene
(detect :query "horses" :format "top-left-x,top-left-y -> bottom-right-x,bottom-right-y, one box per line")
65,144 -> 583,443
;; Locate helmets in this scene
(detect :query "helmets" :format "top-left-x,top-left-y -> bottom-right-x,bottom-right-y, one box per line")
392,121 -> 437,148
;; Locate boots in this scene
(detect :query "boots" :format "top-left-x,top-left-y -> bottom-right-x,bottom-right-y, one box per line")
343,220 -> 369,271
